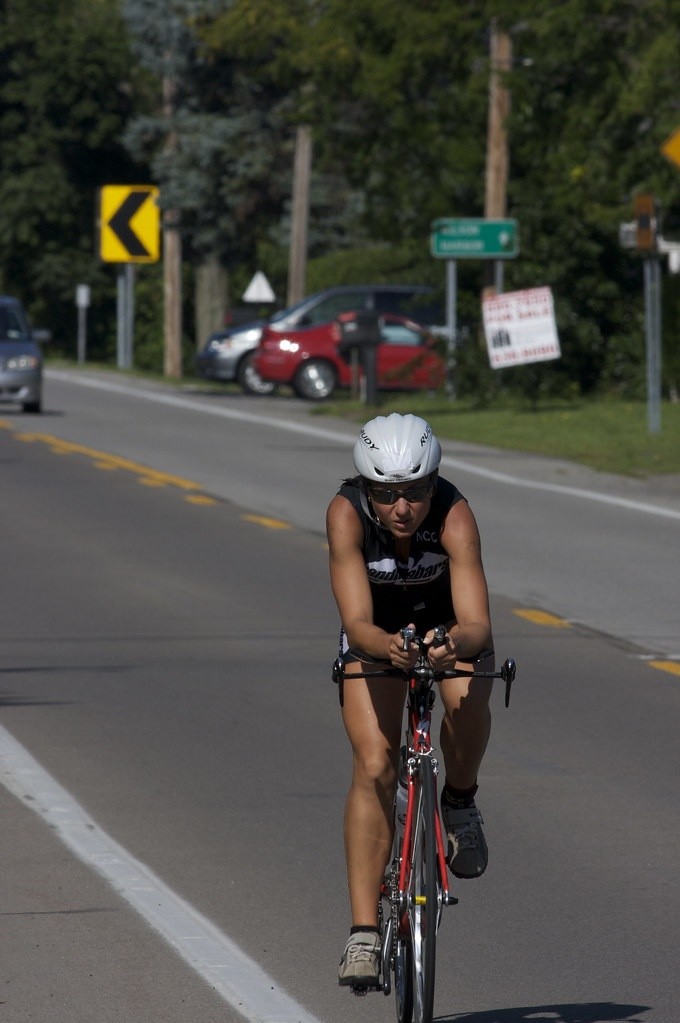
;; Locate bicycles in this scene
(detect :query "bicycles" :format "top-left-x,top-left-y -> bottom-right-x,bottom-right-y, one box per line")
332,625 -> 517,1023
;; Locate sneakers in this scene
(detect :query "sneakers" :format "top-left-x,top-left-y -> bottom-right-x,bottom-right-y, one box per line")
338,931 -> 382,987
440,795 -> 488,878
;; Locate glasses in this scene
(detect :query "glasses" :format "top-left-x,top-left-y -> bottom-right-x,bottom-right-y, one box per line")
368,474 -> 433,504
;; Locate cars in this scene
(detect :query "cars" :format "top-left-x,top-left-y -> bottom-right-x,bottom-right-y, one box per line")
259,313 -> 447,400
0,294 -> 52,414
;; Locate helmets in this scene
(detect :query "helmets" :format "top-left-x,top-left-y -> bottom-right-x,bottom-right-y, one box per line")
353,412 -> 441,483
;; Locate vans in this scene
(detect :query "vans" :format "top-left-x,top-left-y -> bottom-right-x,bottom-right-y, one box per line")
199,286 -> 437,397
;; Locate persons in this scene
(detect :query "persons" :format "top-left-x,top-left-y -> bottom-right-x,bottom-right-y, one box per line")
328,412 -> 495,985
334,309 -> 383,406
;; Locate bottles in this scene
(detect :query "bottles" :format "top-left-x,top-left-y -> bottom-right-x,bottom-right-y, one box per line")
395,768 -> 409,839
